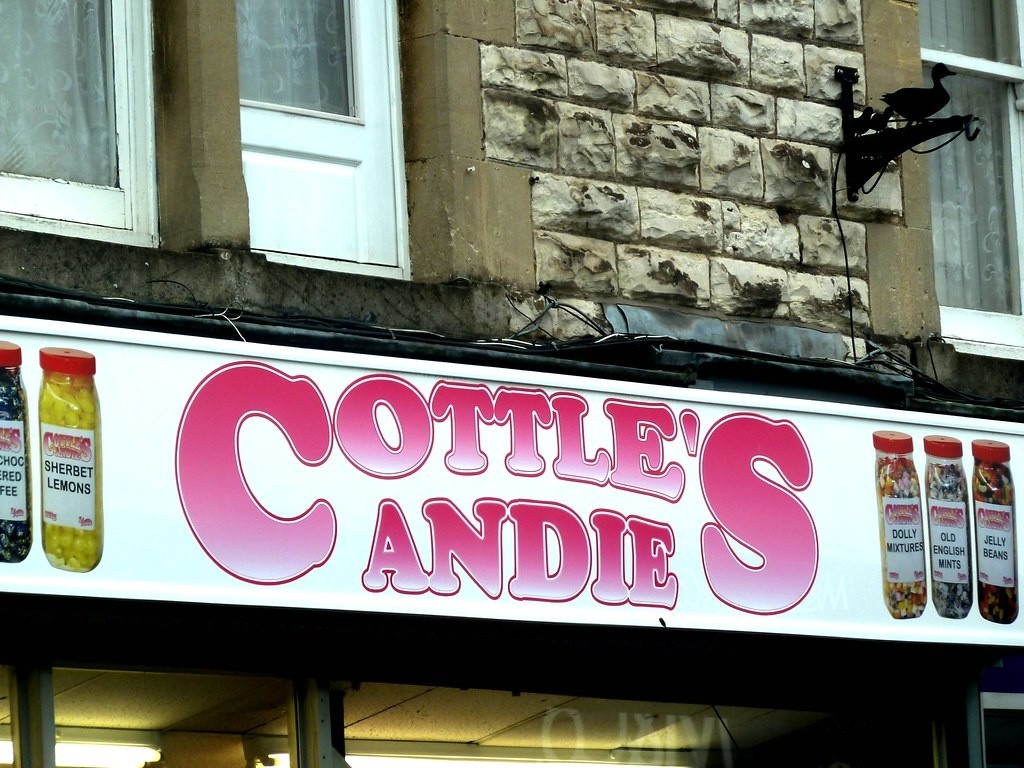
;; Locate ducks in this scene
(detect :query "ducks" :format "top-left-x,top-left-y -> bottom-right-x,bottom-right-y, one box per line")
879,63 -> 957,127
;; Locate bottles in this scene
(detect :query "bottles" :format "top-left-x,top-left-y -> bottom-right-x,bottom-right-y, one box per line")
971,438 -> 1019,625
872,430 -> 927,618
923,435 -> 974,619
0,339 -> 34,564
38,346 -> 104,572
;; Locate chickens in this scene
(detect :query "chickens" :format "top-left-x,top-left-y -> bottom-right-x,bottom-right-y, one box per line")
852,107 -> 875,138
869,106 -> 895,134
